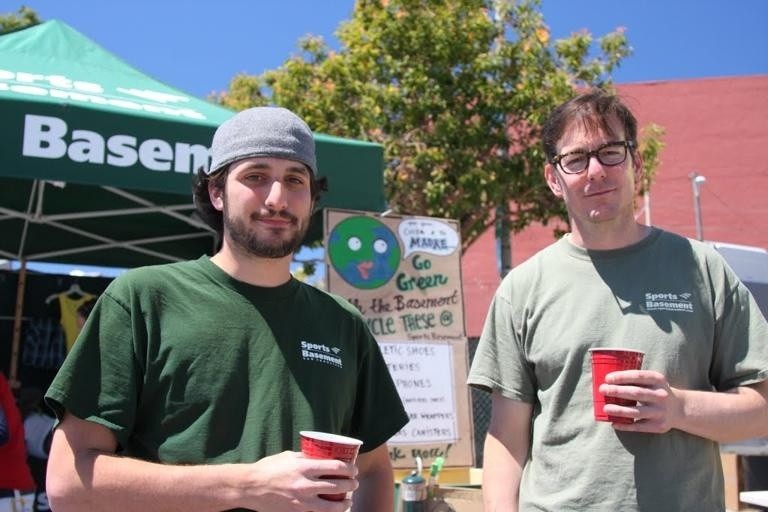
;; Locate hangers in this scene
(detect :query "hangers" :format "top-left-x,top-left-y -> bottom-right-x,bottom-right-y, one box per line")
44,277 -> 97,305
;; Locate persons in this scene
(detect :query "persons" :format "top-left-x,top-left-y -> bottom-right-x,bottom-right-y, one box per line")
44,107 -> 401,511
0,293 -> 100,502
464,88 -> 768,511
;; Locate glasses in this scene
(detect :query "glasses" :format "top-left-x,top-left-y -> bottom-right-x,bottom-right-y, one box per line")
549,140 -> 638,176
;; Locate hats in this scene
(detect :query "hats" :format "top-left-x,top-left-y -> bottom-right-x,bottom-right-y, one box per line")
205,104 -> 319,180
